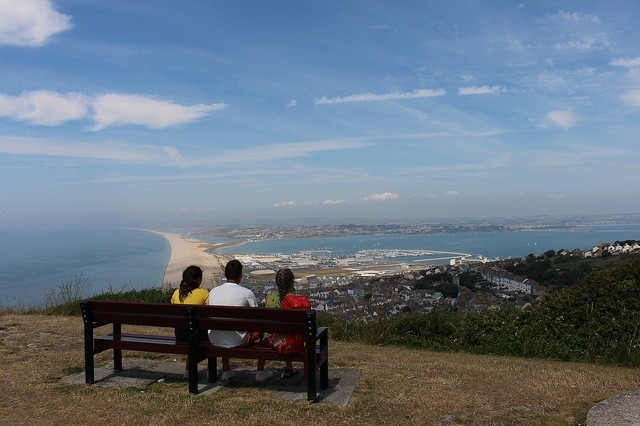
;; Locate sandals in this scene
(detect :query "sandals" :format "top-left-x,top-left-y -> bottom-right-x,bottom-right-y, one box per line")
283,367 -> 299,377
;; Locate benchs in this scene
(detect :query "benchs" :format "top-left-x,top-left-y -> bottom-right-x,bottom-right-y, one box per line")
78,299 -> 329,401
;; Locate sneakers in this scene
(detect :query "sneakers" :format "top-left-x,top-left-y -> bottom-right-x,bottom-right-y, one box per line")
221,369 -> 236,380
256,368 -> 273,380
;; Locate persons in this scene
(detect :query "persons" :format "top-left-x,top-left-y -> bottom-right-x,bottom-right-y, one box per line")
213,260 -> 274,382
264,268 -> 312,380
171,265 -> 220,379
